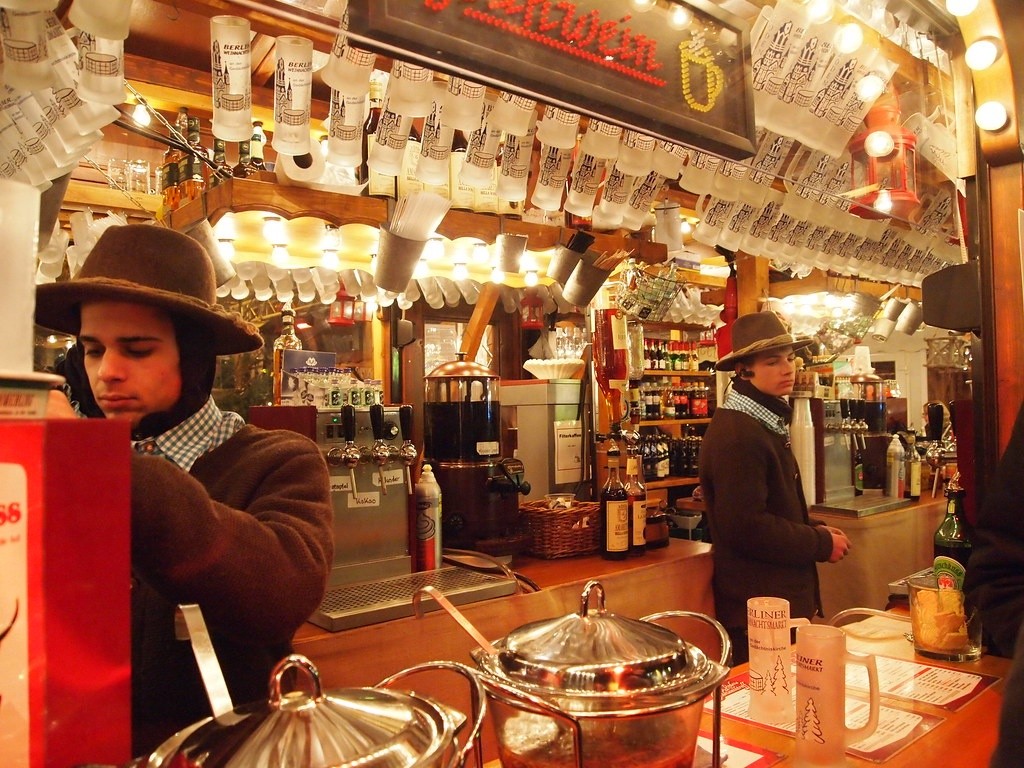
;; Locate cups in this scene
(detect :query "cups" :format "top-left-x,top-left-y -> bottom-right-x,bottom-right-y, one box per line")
789,397 -> 815,510
906,575 -> 983,663
747,597 -> 812,725
855,346 -> 873,374
796,623 -> 880,768
0,0 -> 967,337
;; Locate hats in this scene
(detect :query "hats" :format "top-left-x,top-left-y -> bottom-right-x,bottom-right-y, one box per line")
34,223 -> 264,356
714,311 -> 815,372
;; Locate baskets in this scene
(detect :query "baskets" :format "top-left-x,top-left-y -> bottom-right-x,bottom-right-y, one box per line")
518,499 -> 601,559
616,256 -> 687,325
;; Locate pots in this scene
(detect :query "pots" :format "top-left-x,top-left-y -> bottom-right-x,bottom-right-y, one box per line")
468,579 -> 731,768
77,660 -> 487,768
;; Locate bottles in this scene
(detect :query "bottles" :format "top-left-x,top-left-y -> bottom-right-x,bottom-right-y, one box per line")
273,308 -> 383,407
904,434 -> 922,502
623,446 -> 647,557
629,338 -> 709,482
933,489 -> 982,650
414,465 -> 442,572
884,434 -> 905,500
600,445 -> 630,561
852,442 -> 864,496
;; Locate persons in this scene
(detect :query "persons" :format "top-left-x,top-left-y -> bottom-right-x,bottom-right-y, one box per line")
963,399 -> 1024,768
923,401 -> 955,446
34,225 -> 334,764
698,311 -> 853,669
